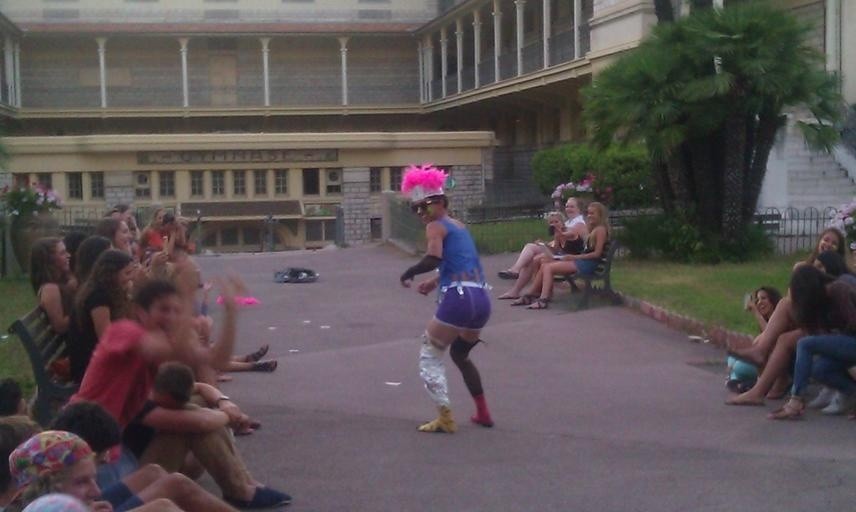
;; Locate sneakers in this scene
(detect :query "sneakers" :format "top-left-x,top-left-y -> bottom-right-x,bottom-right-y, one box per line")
808,387 -> 833,408
226,485 -> 284,510
821,390 -> 850,415
261,485 -> 294,504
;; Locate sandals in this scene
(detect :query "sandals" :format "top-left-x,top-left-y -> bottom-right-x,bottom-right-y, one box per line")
245,344 -> 269,362
497,293 -> 520,299
232,426 -> 255,435
527,298 -> 548,309
510,296 -> 531,306
255,359 -> 278,372
725,385 -> 765,405
497,269 -> 519,279
727,344 -> 765,368
766,398 -> 807,420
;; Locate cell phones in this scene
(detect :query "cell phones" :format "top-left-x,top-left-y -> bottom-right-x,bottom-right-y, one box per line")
163,236 -> 168,255
143,251 -> 151,272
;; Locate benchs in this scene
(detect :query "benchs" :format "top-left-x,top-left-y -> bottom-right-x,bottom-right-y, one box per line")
556,236 -> 624,310
7,303 -> 79,430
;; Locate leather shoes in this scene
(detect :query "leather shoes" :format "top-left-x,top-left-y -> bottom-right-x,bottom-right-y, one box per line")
766,375 -> 793,399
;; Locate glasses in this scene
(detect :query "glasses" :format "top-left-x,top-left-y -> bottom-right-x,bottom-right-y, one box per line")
96,450 -> 111,464
411,199 -> 439,211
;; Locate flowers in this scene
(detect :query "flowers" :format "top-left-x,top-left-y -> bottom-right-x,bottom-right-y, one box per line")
550,171 -> 615,210
0,179 -> 66,220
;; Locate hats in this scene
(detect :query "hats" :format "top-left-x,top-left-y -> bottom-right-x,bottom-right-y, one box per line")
410,185 -> 445,202
2,427 -> 93,512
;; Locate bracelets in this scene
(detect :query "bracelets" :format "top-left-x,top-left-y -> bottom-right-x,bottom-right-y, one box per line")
214,397 -> 231,408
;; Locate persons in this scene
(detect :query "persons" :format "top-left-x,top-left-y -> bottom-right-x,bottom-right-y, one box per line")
400,194 -> 494,434
723,226 -> 856,422
497,196 -> 589,299
534,210 -> 584,301
510,201 -> 611,309
0,202 -> 295,512
